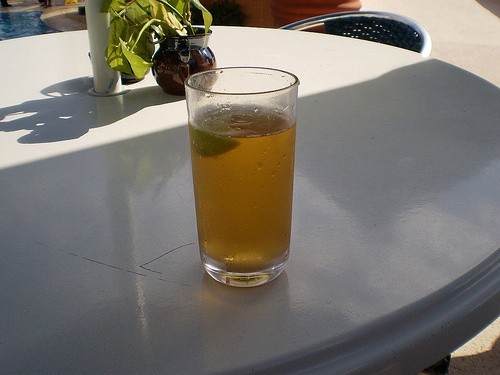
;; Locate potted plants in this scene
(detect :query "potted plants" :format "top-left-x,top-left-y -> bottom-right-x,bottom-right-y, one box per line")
98,0 -> 218,96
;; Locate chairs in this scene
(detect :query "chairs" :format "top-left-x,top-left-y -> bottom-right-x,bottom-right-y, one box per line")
279,10 -> 432,58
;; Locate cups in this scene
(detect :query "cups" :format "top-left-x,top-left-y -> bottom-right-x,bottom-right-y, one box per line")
183,66 -> 300,288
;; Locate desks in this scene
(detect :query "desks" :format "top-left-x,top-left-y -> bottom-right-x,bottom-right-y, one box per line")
1,25 -> 500,375
